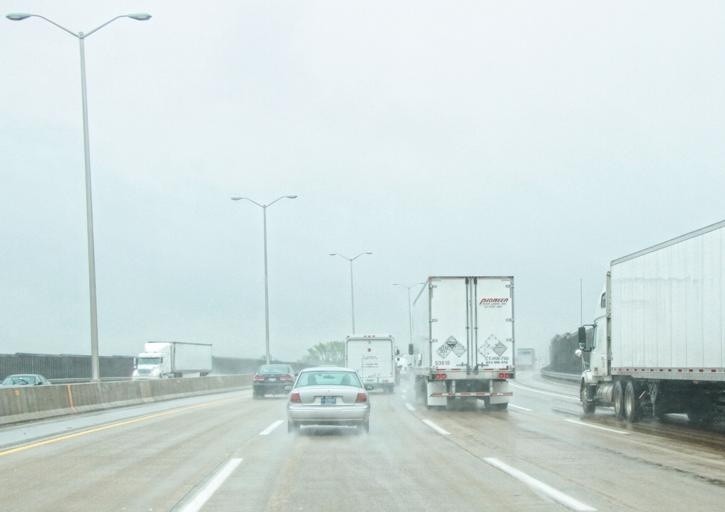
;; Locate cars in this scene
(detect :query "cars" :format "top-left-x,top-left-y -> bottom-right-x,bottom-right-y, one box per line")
252,363 -> 296,400
286,365 -> 374,437
2,373 -> 52,386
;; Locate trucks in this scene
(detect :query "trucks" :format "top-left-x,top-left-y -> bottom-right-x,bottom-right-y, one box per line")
344,333 -> 397,394
131,341 -> 214,382
516,347 -> 535,371
573,220 -> 724,433
407,274 -> 517,411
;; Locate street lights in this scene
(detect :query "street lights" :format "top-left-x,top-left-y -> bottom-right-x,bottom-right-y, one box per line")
328,250 -> 372,336
392,282 -> 425,367
231,194 -> 298,364
5,12 -> 153,385
